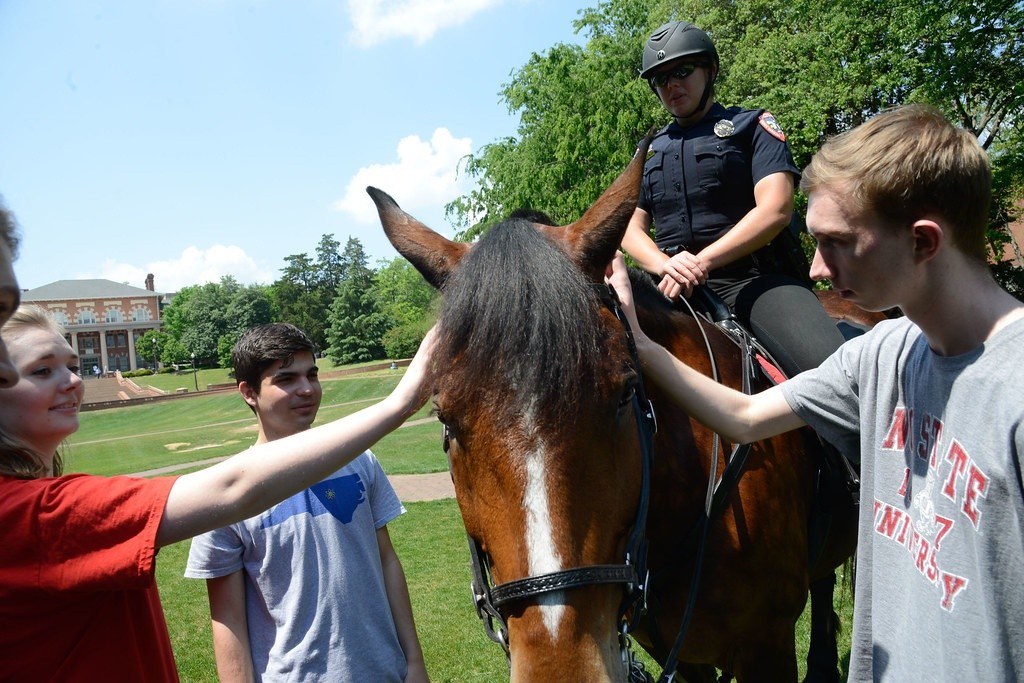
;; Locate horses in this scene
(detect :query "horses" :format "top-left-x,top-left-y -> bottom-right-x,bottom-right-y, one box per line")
366,129 -> 888,683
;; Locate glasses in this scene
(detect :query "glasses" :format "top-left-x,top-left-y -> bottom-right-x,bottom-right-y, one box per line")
649,61 -> 704,87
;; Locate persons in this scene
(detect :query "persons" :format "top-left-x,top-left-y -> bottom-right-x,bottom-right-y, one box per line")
0,304 -> 443,682
0,192 -> 23,390
93,366 -> 101,379
184,323 -> 431,683
606,24 -> 1023,683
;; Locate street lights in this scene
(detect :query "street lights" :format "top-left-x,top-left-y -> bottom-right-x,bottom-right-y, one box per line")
190,352 -> 199,392
152,337 -> 157,374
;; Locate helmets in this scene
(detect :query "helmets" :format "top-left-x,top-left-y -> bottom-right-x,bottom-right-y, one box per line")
637,21 -> 717,79
